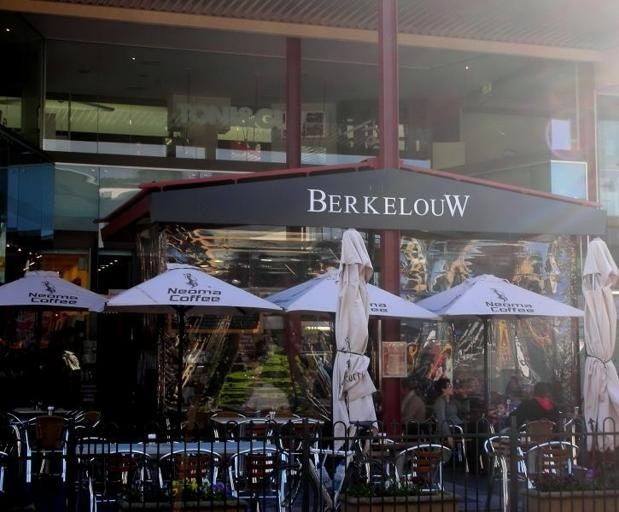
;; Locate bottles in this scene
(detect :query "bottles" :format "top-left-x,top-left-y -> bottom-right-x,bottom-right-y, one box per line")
46,405 -> 56,416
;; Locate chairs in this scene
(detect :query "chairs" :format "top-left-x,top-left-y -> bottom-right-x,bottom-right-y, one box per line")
2,382 -> 600,512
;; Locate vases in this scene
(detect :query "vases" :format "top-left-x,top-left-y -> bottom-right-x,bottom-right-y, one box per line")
517,481 -> 617,512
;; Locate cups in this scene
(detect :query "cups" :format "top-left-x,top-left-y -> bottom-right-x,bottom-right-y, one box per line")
269,412 -> 275,419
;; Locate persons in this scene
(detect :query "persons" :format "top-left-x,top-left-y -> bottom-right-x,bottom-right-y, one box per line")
478,404 -> 506,457
430,378 -> 464,448
495,401 -> 507,417
400,378 -> 424,420
507,380 -> 555,425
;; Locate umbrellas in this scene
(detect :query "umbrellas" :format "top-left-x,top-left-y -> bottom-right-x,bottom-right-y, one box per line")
328,224 -> 378,451
0,269 -> 102,405
581,234 -> 619,451
259,265 -> 444,326
104,262 -> 284,422
416,272 -> 581,406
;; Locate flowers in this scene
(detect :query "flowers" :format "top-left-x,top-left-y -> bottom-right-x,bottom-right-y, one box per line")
525,458 -> 617,492
